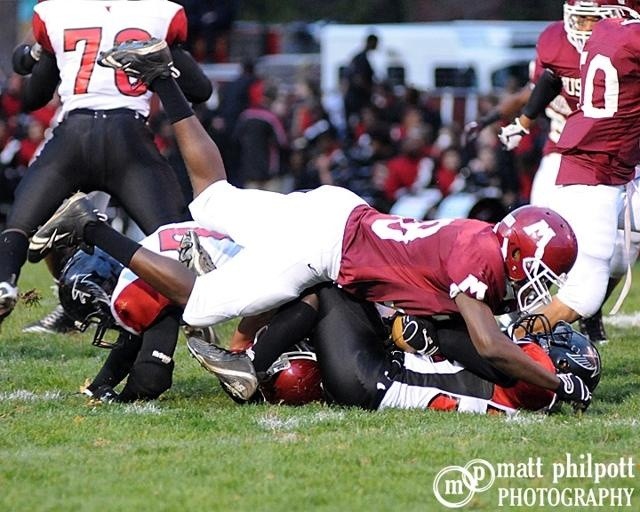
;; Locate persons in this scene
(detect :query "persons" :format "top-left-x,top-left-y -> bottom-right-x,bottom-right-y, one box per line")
27,38 -> 594,409
60,223 -> 246,401
1,0 -> 639,345
183,279 -> 599,416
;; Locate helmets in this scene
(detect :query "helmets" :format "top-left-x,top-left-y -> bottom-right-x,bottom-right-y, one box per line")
260,339 -> 323,408
562,1 -> 640,55
58,246 -> 131,350
495,206 -> 604,411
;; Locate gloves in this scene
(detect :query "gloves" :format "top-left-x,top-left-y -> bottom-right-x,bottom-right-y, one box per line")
496,117 -> 531,153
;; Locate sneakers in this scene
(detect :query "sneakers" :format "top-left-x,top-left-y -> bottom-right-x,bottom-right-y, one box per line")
186,336 -> 260,402
27,192 -> 109,264
23,305 -> 80,337
97,37 -> 182,87
1,282 -> 21,316
